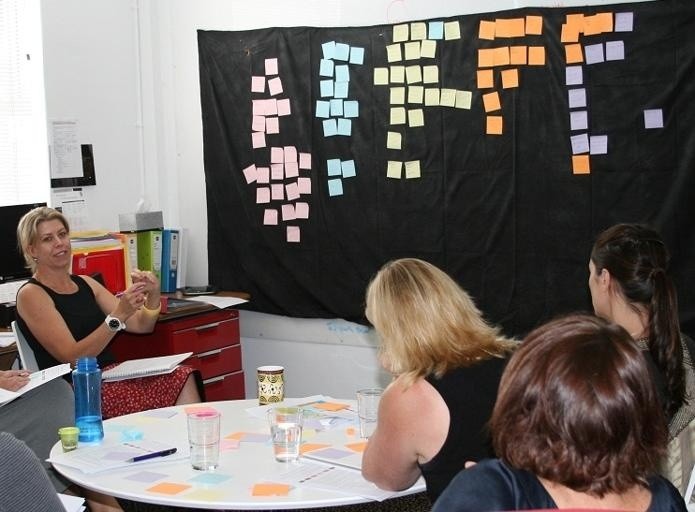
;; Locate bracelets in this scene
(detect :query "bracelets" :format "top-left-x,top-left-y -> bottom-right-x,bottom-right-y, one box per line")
140,299 -> 162,317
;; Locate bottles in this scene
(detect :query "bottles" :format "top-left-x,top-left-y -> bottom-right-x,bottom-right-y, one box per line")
72,356 -> 104,442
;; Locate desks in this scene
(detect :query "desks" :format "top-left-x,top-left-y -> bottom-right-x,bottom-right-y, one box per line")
48,396 -> 429,511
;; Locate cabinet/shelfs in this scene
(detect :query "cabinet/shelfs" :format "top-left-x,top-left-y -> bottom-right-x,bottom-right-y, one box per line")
111,306 -> 245,403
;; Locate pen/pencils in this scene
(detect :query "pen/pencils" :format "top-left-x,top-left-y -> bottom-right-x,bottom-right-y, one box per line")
19,373 -> 25,376
125,448 -> 177,463
115,286 -> 144,297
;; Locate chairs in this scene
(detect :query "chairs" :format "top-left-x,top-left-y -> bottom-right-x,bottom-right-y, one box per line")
11,321 -> 40,375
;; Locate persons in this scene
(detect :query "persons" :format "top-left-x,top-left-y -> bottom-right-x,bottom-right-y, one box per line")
586,223 -> 694,499
15,207 -> 206,421
0,368 -> 126,511
360,255 -> 548,505
429,313 -> 687,511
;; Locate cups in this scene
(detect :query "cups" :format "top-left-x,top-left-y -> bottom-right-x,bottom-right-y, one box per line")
188,410 -> 221,470
257,366 -> 285,405
266,407 -> 302,463
357,388 -> 383,438
57,427 -> 79,451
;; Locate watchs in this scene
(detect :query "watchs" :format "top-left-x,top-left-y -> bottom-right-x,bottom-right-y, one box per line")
104,313 -> 125,331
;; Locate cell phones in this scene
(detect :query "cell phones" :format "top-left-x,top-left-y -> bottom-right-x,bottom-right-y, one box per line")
182,286 -> 215,296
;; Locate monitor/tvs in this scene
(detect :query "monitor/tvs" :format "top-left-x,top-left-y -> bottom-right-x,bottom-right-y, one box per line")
0,202 -> 47,284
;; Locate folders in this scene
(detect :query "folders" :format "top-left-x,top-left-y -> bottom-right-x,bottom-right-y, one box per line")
115,228 -> 179,294
